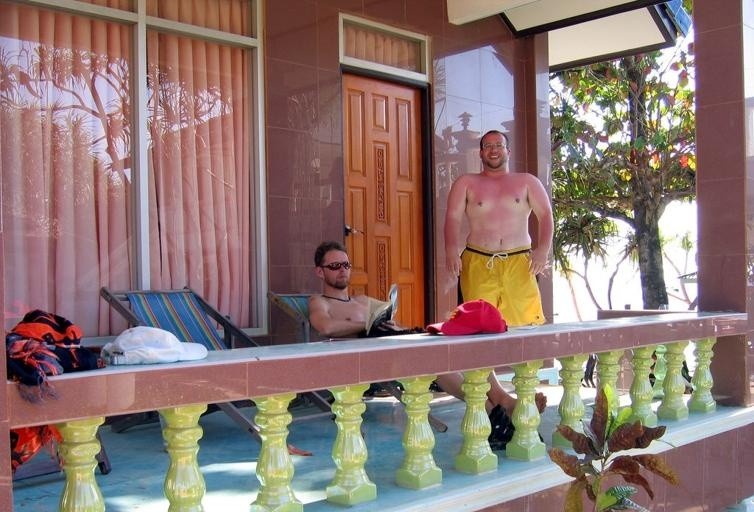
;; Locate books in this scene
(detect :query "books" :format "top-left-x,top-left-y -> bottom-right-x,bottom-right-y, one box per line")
365,284 -> 399,334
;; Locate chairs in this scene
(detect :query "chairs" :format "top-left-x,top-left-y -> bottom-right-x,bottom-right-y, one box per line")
101,285 -> 366,450
267,291 -> 448,433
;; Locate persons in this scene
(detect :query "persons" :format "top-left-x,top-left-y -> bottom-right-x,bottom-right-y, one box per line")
445,131 -> 554,328
309,242 -> 547,427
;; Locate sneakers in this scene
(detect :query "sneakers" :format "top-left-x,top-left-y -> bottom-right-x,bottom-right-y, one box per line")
488,404 -> 544,451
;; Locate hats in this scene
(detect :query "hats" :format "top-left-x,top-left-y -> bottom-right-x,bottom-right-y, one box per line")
101,326 -> 208,364
425,298 -> 508,336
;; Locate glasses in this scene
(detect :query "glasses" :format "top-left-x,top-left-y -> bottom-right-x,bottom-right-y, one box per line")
320,261 -> 350,270
482,142 -> 507,149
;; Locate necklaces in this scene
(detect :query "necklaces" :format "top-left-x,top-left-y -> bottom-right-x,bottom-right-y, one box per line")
322,294 -> 352,303
